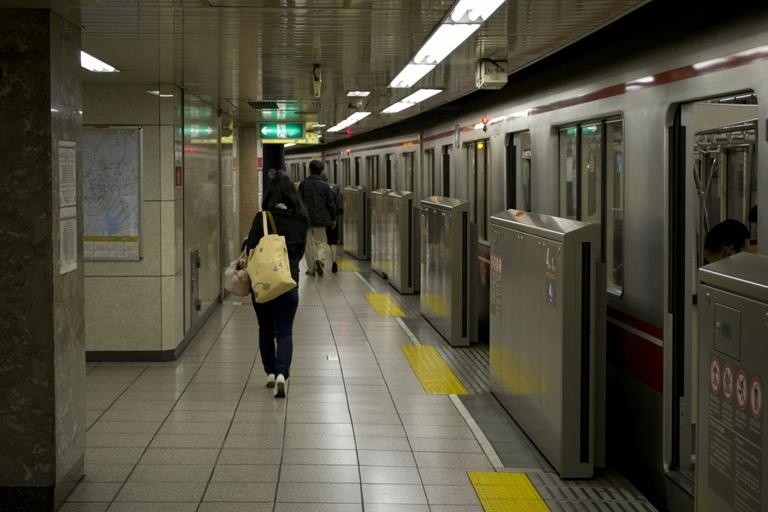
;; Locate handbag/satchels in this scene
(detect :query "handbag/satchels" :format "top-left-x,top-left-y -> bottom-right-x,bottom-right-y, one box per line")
246,211 -> 297,304
223,246 -> 251,297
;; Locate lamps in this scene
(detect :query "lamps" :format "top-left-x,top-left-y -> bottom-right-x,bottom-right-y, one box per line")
386,0 -> 506,89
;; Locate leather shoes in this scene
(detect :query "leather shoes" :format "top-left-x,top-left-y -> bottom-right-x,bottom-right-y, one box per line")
331,261 -> 337,273
272,373 -> 286,398
265,373 -> 276,388
315,260 -> 324,276
305,270 -> 314,276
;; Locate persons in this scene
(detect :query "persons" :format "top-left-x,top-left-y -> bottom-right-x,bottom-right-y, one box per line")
695,218 -> 750,267
319,172 -> 343,273
298,160 -> 335,277
241,174 -> 310,398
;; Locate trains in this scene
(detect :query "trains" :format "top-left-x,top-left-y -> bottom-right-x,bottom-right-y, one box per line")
283,22 -> 768,512
